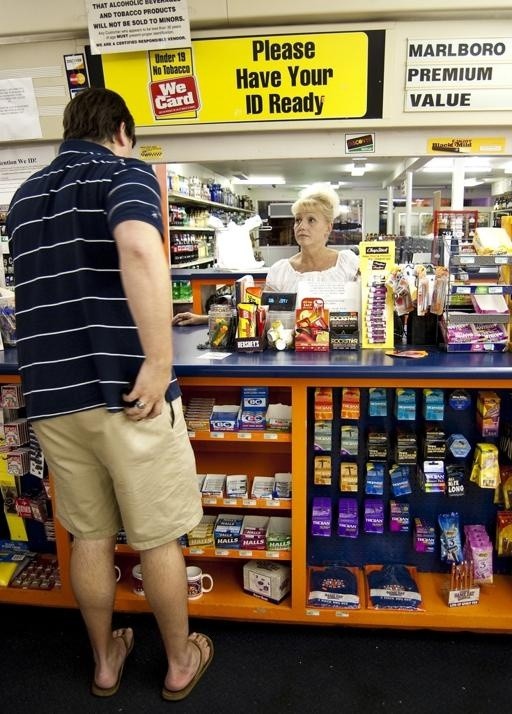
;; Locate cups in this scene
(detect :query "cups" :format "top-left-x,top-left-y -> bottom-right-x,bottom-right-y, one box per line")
131,564 -> 147,596
186,566 -> 214,601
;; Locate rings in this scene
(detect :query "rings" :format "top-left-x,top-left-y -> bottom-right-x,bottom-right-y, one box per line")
136,400 -> 147,411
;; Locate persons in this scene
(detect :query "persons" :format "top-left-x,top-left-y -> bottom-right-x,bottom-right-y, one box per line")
9,88 -> 216,702
171,179 -> 367,326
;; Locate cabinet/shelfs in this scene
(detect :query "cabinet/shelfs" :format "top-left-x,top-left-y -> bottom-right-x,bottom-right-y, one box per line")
156,163 -> 256,304
439,229 -> 510,353
1,375 -> 512,629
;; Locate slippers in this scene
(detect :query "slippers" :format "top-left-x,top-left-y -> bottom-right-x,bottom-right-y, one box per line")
91,627 -> 134,698
163,633 -> 214,701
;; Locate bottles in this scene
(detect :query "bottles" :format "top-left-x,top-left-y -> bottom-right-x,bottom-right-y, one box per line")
363,232 -> 399,241
169,173 -> 257,301
207,304 -> 229,347
